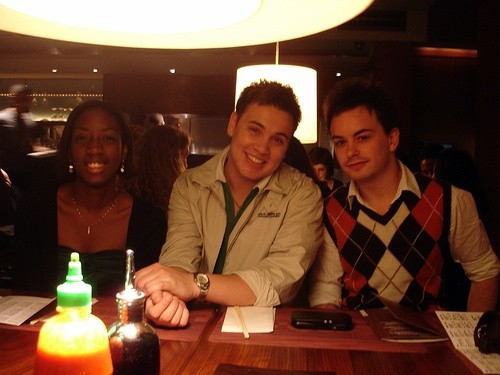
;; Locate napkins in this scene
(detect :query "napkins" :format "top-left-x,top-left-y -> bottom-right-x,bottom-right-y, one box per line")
221,306 -> 276,333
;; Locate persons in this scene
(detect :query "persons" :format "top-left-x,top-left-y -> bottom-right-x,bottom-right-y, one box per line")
0,78 -> 500,329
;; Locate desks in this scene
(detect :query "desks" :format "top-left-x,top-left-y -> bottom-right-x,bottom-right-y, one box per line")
0,290 -> 500,375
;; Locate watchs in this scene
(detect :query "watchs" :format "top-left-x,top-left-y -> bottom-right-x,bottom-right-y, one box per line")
192,273 -> 210,304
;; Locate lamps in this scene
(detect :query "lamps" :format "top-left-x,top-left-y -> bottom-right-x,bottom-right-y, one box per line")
234,42 -> 318,143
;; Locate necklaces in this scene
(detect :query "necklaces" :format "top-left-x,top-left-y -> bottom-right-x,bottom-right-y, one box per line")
71,192 -> 118,235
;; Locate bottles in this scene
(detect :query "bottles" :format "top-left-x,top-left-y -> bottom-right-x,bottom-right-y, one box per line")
34,252 -> 113,375
107,250 -> 161,375
